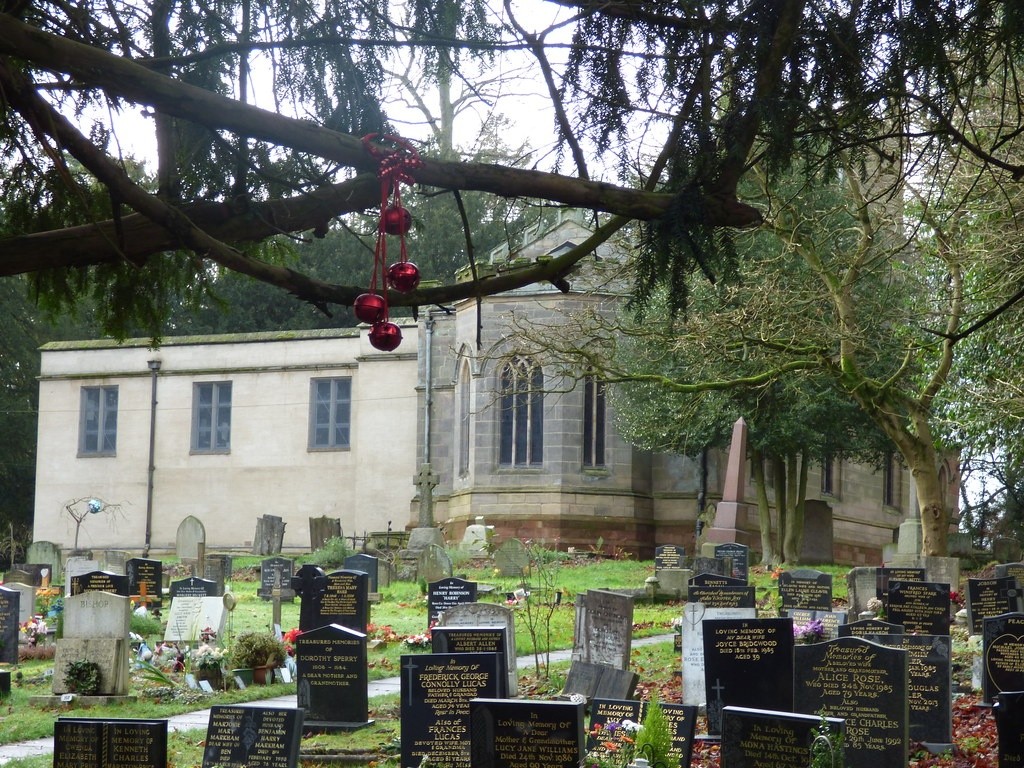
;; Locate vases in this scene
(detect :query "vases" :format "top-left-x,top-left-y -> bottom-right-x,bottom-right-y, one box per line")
195,669 -> 223,691
31,641 -> 39,648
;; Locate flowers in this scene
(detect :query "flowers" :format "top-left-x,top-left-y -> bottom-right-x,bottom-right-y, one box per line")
586,719 -> 640,762
132,640 -> 230,671
792,620 -> 825,644
283,628 -> 304,658
19,613 -> 52,642
867,596 -> 888,618
949,591 -> 966,603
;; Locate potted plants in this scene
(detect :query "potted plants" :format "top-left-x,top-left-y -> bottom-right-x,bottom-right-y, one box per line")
232,631 -> 288,690
